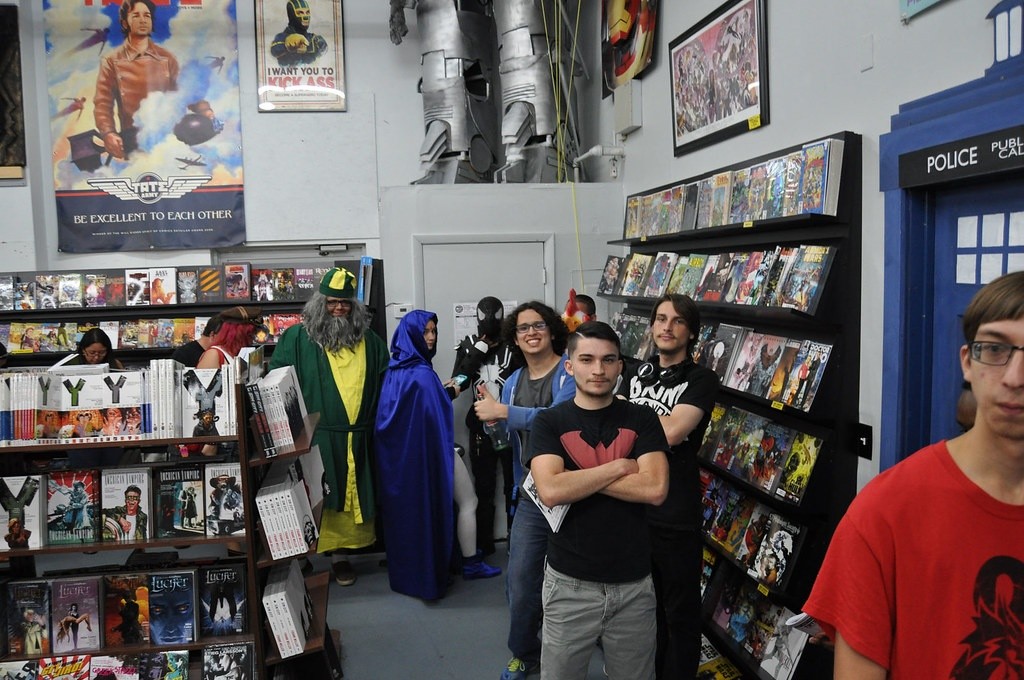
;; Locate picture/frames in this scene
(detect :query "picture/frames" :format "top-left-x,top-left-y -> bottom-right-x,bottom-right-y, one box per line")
668,0 -> 770,157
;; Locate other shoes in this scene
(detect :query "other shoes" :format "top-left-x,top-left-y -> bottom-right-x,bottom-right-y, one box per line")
331,559 -> 356,585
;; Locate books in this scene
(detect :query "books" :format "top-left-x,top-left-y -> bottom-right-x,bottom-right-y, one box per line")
0,454 -> 246,551
255,444 -> 331,560
597,138 -> 845,360
263,559 -> 345,680
0,359 -> 252,447
0,256 -> 382,353
247,365 -> 307,457
0,556 -> 259,680
693,322 -> 834,680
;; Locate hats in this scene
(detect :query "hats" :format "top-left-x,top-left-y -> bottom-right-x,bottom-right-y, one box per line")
218,307 -> 263,324
319,267 -> 357,298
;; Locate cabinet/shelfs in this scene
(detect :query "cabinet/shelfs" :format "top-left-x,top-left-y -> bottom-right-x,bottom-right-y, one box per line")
0,256 -> 386,680
596,130 -> 863,679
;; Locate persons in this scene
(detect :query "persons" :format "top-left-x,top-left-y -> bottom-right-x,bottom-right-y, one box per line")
374,310 -> 502,601
67,328 -> 124,469
802,271 -> 1024,680
269,267 -> 389,585
565,294 -> 597,321
197,306 -> 264,555
615,294 -> 715,680
475,302 -> 578,680
142,317 -> 222,461
527,322 -> 672,680
451,296 -> 525,559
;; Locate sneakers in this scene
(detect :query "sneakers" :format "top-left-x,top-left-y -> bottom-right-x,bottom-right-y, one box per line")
460,550 -> 502,579
501,655 -> 539,680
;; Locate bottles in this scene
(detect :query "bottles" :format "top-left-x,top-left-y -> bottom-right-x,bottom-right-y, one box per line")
476,393 -> 508,450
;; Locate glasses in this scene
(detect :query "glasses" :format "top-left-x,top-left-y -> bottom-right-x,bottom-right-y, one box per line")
325,300 -> 352,307
968,341 -> 1024,366
514,322 -> 551,332
84,349 -> 107,357
